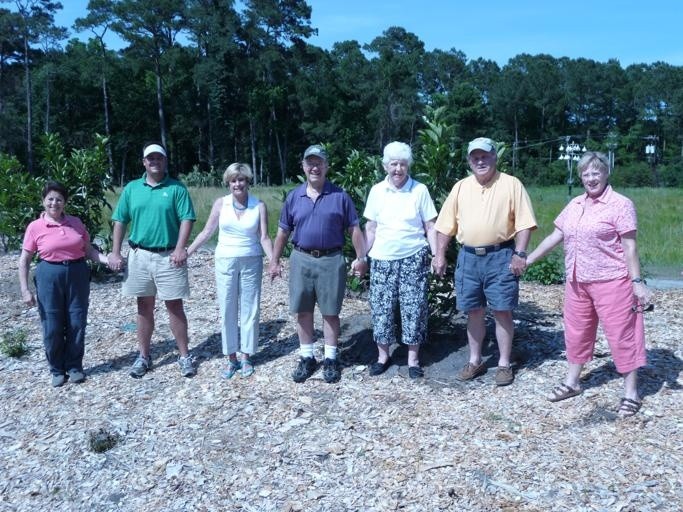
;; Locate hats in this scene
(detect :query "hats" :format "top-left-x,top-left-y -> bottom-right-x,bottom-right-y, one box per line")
143,144 -> 167,158
468,136 -> 496,155
304,145 -> 328,159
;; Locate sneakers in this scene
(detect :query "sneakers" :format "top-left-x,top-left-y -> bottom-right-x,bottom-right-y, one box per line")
323,357 -> 341,383
367,361 -> 516,386
52,372 -> 65,388
69,370 -> 83,383
292,356 -> 317,383
131,354 -> 254,378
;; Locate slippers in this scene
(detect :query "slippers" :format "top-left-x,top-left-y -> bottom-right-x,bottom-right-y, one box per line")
618,397 -> 641,417
547,382 -> 584,402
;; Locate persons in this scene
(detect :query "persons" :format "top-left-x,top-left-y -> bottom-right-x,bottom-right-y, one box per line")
352,139 -> 445,379
172,163 -> 281,376
432,136 -> 536,388
18,181 -> 121,388
103,142 -> 197,379
513,151 -> 657,419
272,144 -> 370,385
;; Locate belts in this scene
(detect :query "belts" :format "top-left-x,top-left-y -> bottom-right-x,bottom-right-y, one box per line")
294,245 -> 342,257
464,240 -> 514,253
135,244 -> 174,253
46,257 -> 86,265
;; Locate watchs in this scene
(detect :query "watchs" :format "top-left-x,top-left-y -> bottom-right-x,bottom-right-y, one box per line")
632,277 -> 642,283
356,255 -> 369,265
513,249 -> 527,259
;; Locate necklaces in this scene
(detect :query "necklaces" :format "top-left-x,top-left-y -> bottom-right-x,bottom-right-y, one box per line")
233,197 -> 248,210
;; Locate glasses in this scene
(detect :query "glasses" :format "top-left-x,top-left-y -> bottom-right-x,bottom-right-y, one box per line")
632,304 -> 655,313
